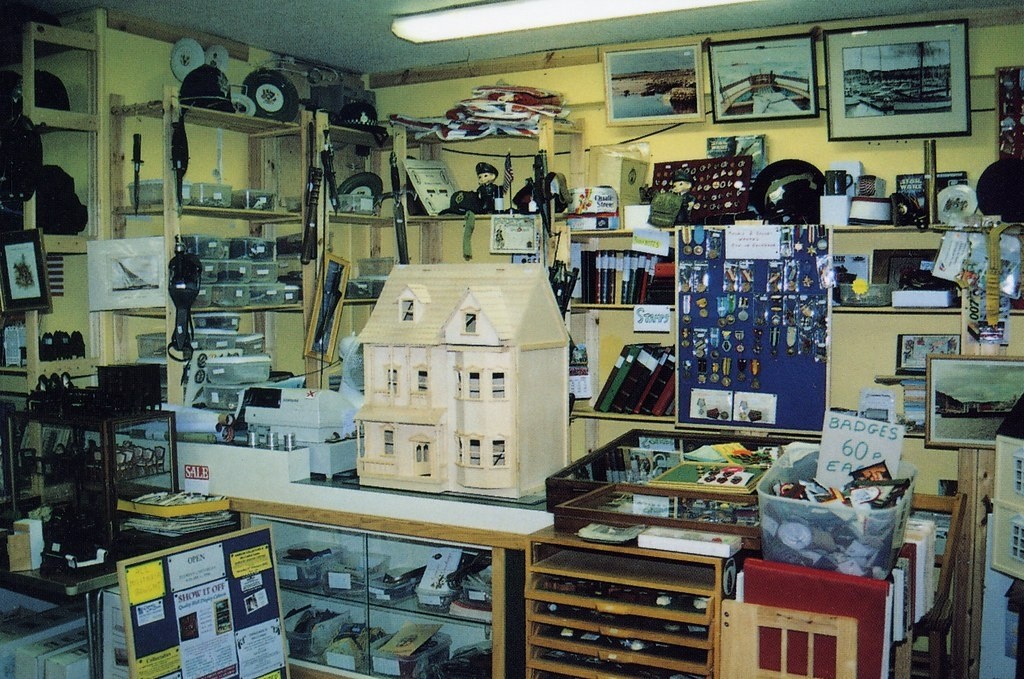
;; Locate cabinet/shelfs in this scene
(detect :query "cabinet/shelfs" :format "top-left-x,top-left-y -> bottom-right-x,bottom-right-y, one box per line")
227,497 -> 527,679
7,412 -> 178,545
103,82 -> 315,392
370,112 -> 585,267
523,524 -> 729,679
246,111 -> 407,394
557,221 -> 963,315
0,21 -> 99,396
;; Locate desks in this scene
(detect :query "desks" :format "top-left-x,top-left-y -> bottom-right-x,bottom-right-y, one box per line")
0,515 -> 241,679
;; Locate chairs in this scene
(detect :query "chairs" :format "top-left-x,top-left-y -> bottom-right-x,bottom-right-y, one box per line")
909,490 -> 968,679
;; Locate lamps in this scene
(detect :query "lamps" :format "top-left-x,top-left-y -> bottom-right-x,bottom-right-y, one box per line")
389,0 -> 757,46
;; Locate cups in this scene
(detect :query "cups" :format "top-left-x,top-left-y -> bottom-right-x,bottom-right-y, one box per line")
825,170 -> 852,195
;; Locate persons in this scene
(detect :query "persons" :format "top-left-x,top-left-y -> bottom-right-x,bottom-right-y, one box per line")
672,170 -> 697,227
476,162 -> 504,214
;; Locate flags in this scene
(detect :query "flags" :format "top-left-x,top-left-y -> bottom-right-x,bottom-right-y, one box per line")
504,153 -> 513,194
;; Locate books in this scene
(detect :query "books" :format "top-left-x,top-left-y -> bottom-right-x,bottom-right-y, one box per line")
570,242 -> 675,304
875,369 -> 926,426
605,437 -> 680,482
0,605 -> 90,679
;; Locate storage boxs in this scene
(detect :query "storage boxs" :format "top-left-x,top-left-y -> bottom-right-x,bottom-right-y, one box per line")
551,484 -> 761,553
272,539 -> 493,614
837,280 -> 958,309
0,585 -> 130,679
127,175 -> 394,409
4,323 -> 27,368
361,630 -> 453,679
560,142 -> 650,232
755,441 -> 919,581
546,427 -> 823,515
284,605 -> 350,660
7,518 -> 46,572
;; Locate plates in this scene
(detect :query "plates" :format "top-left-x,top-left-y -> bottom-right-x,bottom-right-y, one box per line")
936,184 -> 978,223
170,38 -> 204,82
339,172 -> 382,206
230,93 -> 256,116
243,68 -> 299,122
204,44 -> 229,71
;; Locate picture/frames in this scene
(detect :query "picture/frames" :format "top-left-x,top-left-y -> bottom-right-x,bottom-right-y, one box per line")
924,353 -> 1024,451
0,228 -> 53,316
490,214 -> 538,255
301,251 -> 352,362
402,158 -> 460,216
600,19 -> 972,179
86,236 -> 165,312
896,333 -> 962,372
